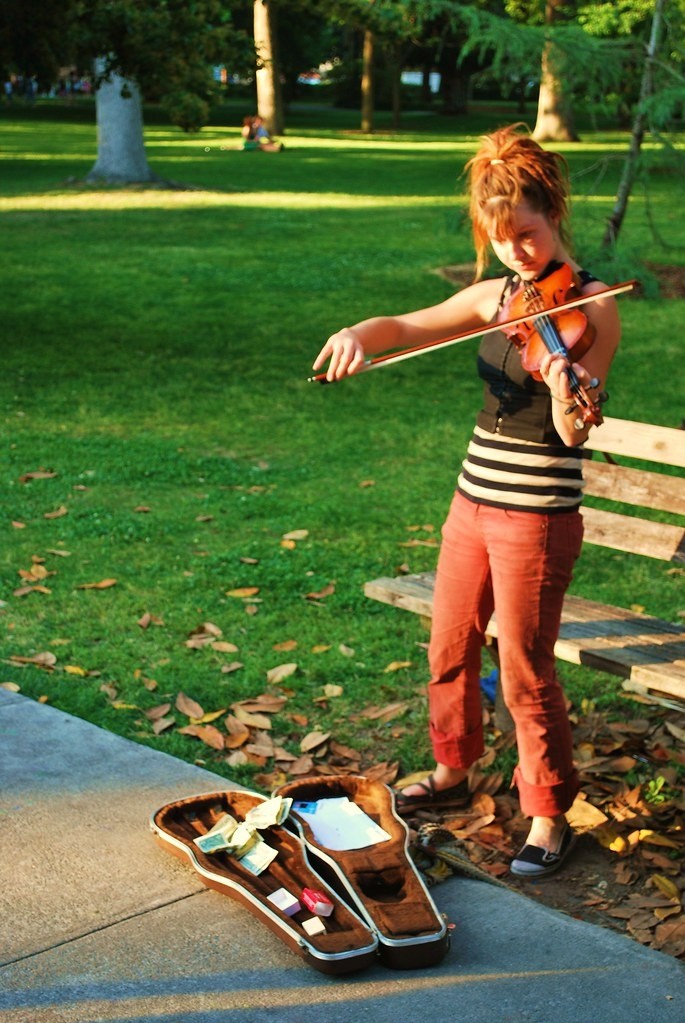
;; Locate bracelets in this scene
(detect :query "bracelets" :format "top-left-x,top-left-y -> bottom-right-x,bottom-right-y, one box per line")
550,393 -> 575,405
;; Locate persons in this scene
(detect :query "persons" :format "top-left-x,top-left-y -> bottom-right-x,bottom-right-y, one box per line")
238,118 -> 283,152
313,122 -> 620,877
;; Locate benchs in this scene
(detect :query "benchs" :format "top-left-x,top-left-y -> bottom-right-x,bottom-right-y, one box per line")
361,414 -> 685,733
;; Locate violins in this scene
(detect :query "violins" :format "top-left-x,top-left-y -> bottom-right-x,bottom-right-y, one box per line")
497,262 -> 609,430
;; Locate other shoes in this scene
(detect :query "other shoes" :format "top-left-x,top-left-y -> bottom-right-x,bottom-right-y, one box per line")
392,775 -> 472,815
510,822 -> 573,875
